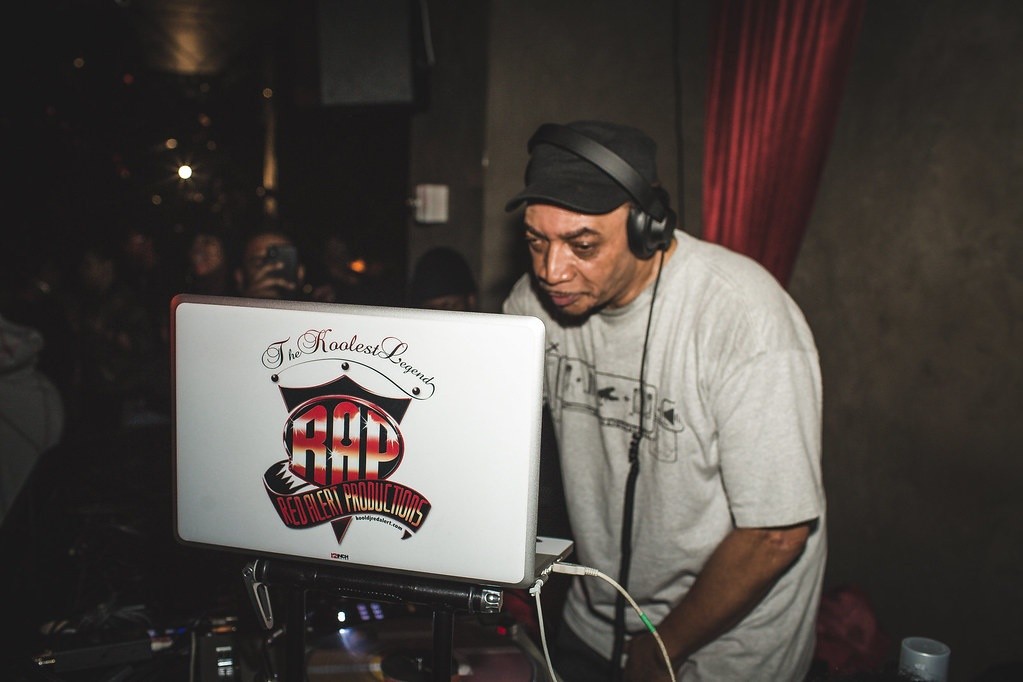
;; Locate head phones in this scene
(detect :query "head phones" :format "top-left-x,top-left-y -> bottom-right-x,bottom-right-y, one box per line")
527,121 -> 676,263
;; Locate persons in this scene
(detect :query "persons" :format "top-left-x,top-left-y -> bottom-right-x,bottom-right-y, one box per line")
500,119 -> 828,682
1,157 -> 480,563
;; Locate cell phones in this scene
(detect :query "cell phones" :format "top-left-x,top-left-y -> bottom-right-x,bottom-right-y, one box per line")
262,243 -> 301,300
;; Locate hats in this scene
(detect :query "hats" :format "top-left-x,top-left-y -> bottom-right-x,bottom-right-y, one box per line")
504,120 -> 659,214
410,247 -> 477,300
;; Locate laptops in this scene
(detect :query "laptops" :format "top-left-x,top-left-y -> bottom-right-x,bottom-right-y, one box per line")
170,293 -> 575,591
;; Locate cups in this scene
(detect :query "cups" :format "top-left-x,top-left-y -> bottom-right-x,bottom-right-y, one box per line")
897,637 -> 950,682
